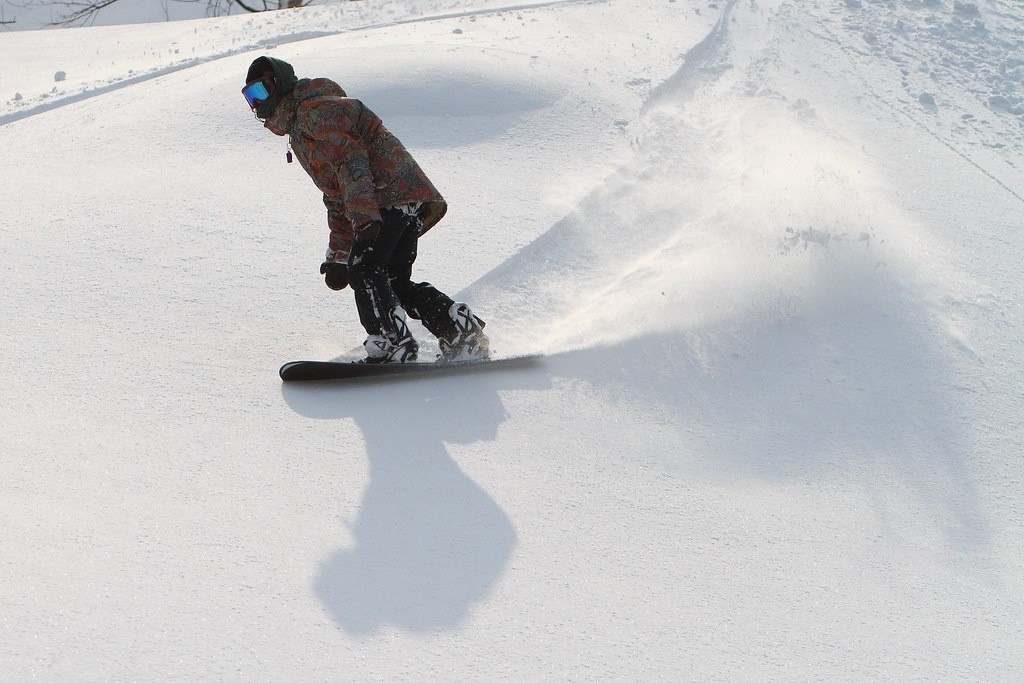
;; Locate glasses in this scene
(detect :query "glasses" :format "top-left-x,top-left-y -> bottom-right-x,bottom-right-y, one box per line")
241,75 -> 277,111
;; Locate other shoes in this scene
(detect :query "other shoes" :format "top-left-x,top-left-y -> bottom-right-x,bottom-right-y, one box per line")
434,303 -> 490,362
355,306 -> 420,364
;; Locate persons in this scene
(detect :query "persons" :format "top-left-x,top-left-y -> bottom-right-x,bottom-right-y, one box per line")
246,56 -> 490,363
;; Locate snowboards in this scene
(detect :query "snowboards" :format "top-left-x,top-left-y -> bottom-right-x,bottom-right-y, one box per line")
276,352 -> 544,382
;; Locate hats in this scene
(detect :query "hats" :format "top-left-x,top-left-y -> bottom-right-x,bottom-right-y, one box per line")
246,55 -> 298,119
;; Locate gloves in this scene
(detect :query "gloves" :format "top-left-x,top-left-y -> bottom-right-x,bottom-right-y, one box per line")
320,261 -> 349,291
360,222 -> 379,242
